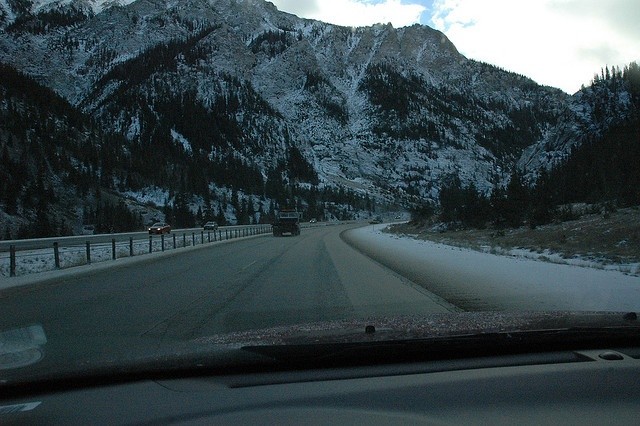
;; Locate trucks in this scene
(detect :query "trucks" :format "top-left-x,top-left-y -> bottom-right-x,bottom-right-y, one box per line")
272,207 -> 301,237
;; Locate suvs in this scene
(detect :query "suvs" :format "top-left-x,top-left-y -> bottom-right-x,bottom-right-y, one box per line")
204,221 -> 219,230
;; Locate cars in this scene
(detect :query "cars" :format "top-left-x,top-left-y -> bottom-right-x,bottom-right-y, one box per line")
309,218 -> 317,223
149,222 -> 171,234
